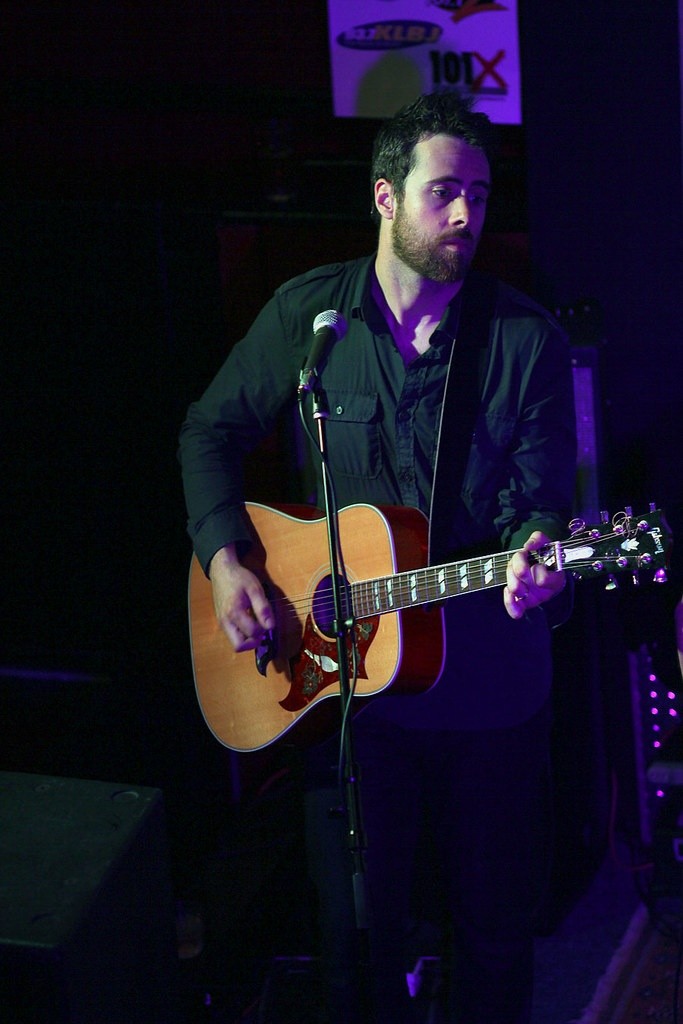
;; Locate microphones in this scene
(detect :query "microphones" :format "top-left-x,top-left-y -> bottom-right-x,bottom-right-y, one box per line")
298,309 -> 348,403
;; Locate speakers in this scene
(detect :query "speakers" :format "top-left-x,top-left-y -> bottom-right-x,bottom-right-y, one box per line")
0,771 -> 188,1024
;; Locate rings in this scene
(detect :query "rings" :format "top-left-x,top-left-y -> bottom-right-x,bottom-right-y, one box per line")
515,594 -> 528,602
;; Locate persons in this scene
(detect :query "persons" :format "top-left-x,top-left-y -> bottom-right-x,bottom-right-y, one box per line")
179,90 -> 577,1024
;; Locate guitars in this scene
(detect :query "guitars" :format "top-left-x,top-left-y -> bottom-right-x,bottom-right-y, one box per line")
186,502 -> 674,755
